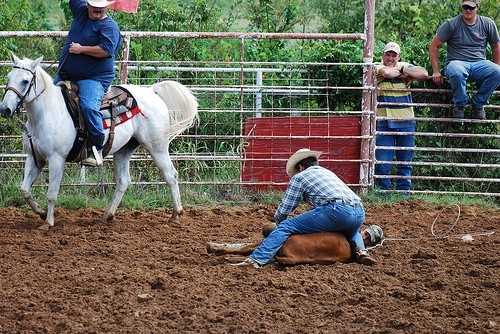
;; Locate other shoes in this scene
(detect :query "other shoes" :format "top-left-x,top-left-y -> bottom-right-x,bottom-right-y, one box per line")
360,256 -> 377,266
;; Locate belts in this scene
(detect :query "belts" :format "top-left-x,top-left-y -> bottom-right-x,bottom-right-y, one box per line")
332,200 -> 361,206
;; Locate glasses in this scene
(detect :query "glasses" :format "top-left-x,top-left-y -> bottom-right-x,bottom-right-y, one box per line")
462,5 -> 475,10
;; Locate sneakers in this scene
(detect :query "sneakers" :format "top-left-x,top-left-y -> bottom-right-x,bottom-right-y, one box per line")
82,150 -> 103,166
474,104 -> 485,118
452,102 -> 465,118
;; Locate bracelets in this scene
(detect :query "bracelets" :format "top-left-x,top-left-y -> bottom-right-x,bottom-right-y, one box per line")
401,66 -> 404,73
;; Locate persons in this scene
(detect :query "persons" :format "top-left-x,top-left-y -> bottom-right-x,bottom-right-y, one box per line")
53,0 -> 121,167
360,224 -> 383,246
430,0 -> 500,119
240,148 -> 377,267
375,42 -> 428,195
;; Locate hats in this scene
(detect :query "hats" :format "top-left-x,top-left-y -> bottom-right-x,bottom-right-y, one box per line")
365,225 -> 383,243
383,42 -> 400,54
461,0 -> 480,8
86,0 -> 108,7
286,149 -> 323,179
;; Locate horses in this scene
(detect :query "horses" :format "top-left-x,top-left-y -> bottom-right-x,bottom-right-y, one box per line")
205,211 -> 350,268
0,50 -> 199,231
